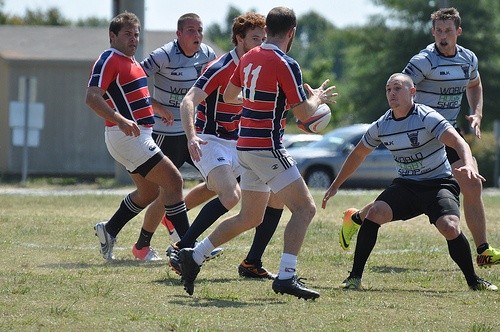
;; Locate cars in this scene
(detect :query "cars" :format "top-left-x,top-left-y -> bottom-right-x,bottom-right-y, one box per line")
282,124 -> 402,186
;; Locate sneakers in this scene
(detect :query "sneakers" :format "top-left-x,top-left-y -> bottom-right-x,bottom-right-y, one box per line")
339,276 -> 361,289
165,241 -> 179,274
161,214 -> 180,242
272,275 -> 320,301
238,259 -> 277,279
94,221 -> 119,260
467,277 -> 499,291
338,208 -> 361,251
476,244 -> 500,269
132,242 -> 162,261
205,248 -> 223,261
175,248 -> 202,296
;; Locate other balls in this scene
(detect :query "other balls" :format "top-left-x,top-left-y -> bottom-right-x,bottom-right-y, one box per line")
296,103 -> 332,134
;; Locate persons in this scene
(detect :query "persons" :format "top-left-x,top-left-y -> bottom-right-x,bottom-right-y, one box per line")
177,6 -> 338,303
321,73 -> 498,294
165,12 -> 280,280
130,13 -> 218,261
84,13 -> 224,264
339,6 -> 500,269
